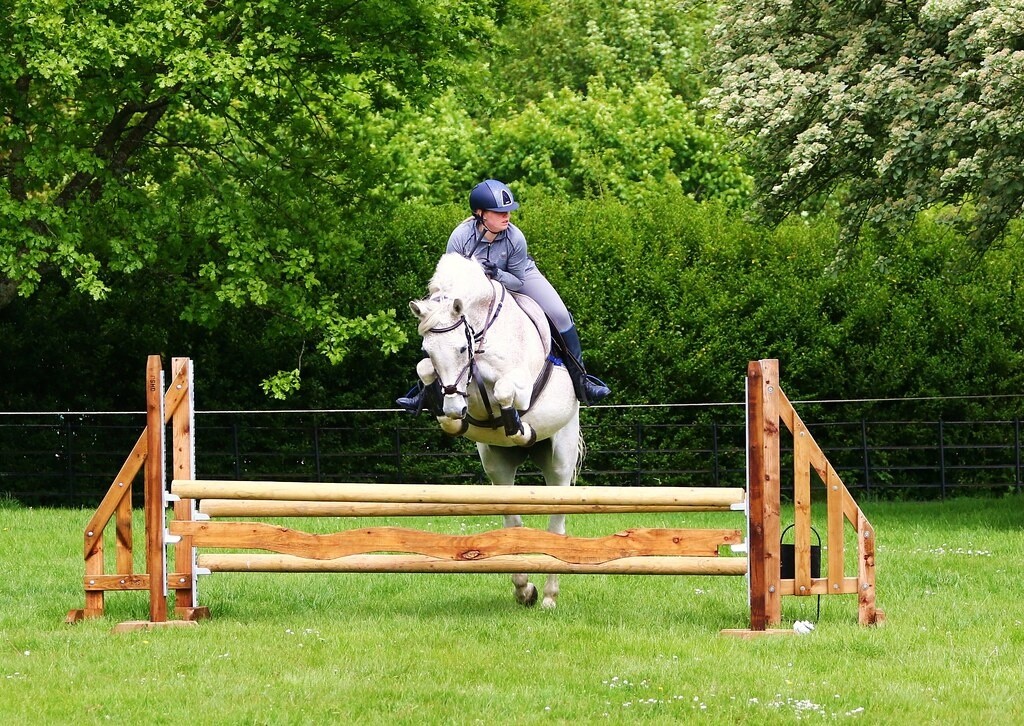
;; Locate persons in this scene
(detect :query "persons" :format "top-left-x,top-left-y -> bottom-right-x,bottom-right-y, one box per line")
396,180 -> 612,408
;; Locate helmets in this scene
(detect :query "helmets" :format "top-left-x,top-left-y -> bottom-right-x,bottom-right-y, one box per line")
470,180 -> 519,212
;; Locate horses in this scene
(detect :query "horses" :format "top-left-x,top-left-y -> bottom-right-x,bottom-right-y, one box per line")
408,251 -> 587,610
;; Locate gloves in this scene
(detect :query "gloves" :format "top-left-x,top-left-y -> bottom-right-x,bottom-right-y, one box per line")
482,260 -> 498,279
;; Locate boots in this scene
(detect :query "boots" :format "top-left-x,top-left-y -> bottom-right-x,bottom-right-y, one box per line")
395,381 -> 441,411
559,324 -> 612,403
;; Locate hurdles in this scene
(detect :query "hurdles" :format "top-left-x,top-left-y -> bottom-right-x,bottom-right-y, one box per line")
66,355 -> 884,638
